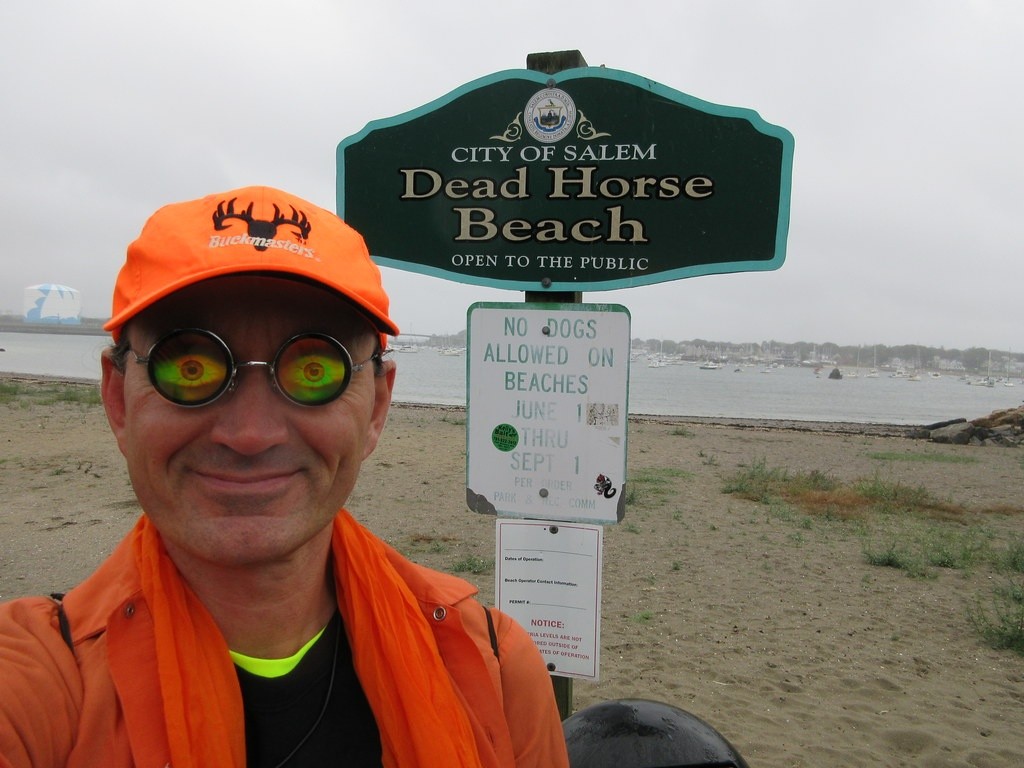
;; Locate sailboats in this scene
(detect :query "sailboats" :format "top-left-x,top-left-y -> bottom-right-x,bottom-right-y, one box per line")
844,345 -> 925,381
699,349 -> 725,369
630,338 -> 673,368
394,338 -> 464,355
962,350 -> 1014,388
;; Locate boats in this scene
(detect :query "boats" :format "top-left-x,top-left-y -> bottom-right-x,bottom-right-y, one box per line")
814,368 -> 842,380
735,363 -> 785,374
929,371 -> 939,377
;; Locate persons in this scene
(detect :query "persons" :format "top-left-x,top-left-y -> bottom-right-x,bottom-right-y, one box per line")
0,185 -> 569,768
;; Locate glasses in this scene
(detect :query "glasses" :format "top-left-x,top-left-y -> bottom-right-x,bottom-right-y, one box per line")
116,326 -> 395,407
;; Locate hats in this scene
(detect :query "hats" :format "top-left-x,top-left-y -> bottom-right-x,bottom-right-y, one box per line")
100,186 -> 398,338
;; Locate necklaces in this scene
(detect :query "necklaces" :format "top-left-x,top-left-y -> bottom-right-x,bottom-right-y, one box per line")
272,623 -> 341,768
